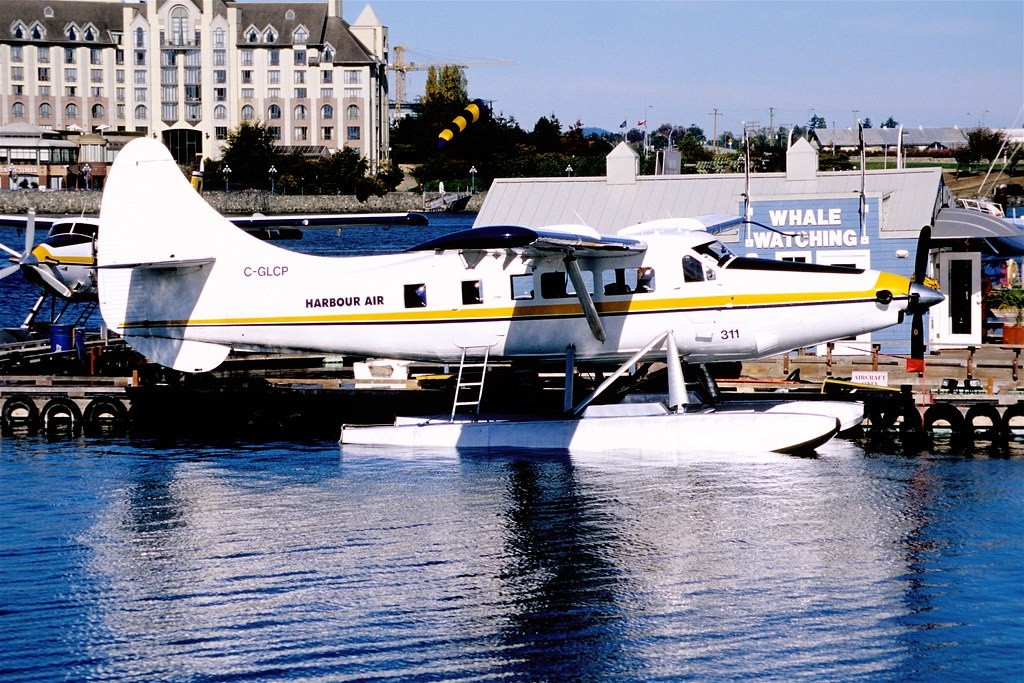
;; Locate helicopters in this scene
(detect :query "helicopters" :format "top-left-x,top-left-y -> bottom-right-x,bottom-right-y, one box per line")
0,178 -> 431,303
91,133 -> 945,453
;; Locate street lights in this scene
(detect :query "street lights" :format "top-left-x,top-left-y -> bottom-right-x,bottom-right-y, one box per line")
468,166 -> 477,190
565,165 -> 573,177
645,104 -> 654,156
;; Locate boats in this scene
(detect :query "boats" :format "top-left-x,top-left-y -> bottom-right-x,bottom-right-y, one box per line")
422,190 -> 473,213
